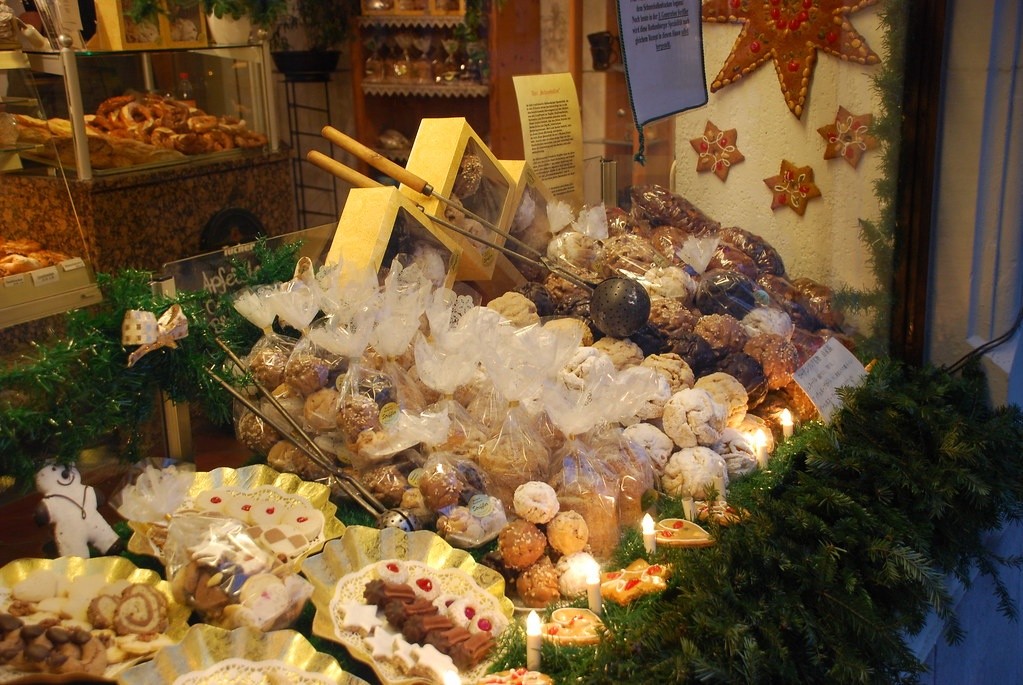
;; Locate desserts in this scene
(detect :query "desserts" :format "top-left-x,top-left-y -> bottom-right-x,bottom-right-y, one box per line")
0,234 -> 779,685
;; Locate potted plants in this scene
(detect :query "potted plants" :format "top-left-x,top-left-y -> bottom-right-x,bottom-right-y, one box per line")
124,0 -> 362,82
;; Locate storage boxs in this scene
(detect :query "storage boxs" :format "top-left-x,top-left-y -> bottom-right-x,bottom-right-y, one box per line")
10,36 -> 280,177
325,116 -> 565,300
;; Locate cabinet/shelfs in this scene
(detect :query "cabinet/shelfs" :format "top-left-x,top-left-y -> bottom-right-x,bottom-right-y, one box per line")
348,0 -> 542,189
0,49 -> 104,330
569,0 -> 675,213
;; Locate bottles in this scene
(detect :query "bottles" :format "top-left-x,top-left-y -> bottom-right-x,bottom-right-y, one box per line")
177,72 -> 196,108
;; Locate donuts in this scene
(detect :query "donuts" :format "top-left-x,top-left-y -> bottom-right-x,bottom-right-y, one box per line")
89,92 -> 267,154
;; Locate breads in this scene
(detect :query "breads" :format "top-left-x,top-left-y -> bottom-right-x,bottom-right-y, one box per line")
596,184 -> 861,364
